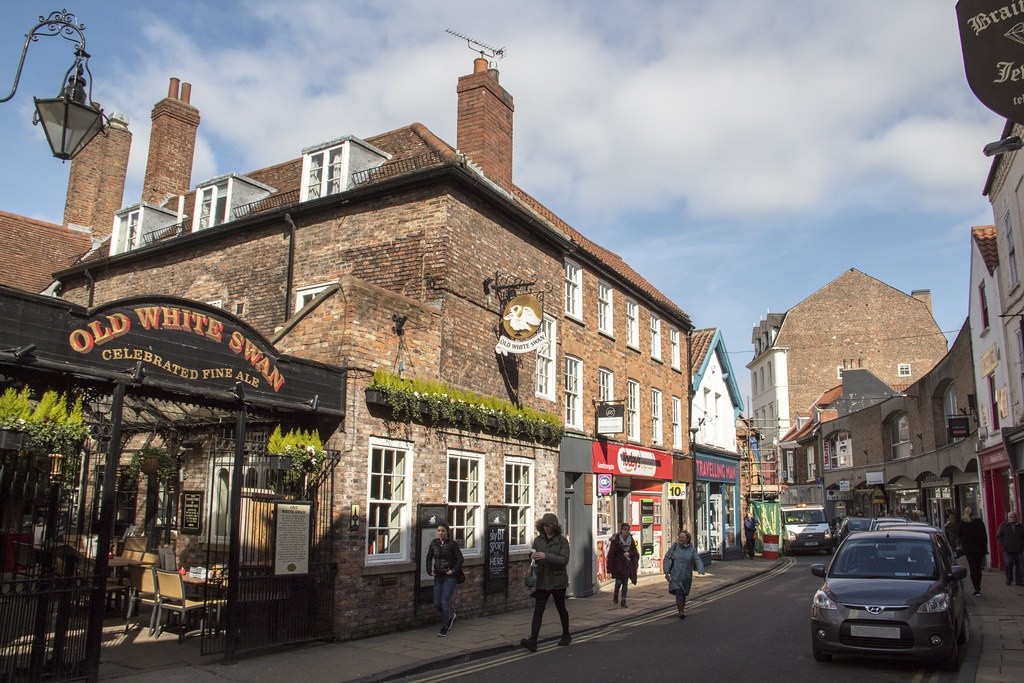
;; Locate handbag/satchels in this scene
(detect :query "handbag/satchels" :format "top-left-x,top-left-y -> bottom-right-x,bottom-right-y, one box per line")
525,550 -> 537,588
455,567 -> 465,584
753,531 -> 758,540
668,558 -> 674,574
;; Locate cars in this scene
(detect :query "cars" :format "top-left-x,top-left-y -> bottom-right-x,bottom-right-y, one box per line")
809,531 -> 969,671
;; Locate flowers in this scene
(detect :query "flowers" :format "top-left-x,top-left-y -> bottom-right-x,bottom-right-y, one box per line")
284,445 -> 328,474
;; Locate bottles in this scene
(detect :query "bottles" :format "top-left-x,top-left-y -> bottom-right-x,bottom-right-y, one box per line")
208,568 -> 222,584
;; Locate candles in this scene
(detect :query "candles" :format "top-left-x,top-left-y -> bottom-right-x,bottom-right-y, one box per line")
178,566 -> 186,576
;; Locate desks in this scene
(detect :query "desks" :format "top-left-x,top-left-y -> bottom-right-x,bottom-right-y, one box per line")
12,541 -> 64,550
180,571 -> 227,637
70,544 -> 156,613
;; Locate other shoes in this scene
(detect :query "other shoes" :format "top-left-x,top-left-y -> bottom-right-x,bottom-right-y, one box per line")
678,610 -> 686,617
621,599 -> 628,607
520,638 -> 537,652
1016,581 -> 1024,586
613,593 -> 618,603
1005,581 -> 1011,585
749,555 -> 754,559
972,591 -> 980,597
558,634 -> 572,646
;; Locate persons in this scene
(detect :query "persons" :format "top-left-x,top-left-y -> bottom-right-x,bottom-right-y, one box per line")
520,513 -> 572,651
606,523 -> 640,608
826,506 -> 1024,597
427,522 -> 465,637
663,531 -> 706,620
744,512 -> 760,559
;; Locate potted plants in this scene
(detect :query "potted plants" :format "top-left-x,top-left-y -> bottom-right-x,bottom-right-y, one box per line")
140,447 -> 158,474
264,426 -> 324,471
365,366 -> 565,443
0,384 -> 88,457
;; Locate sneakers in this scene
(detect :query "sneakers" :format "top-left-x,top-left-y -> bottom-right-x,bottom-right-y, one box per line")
448,613 -> 456,630
437,628 -> 449,636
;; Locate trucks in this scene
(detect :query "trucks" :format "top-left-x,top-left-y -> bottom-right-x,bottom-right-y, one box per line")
780,505 -> 833,555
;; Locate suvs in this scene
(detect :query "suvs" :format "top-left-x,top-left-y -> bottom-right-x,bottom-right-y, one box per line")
835,514 -> 964,562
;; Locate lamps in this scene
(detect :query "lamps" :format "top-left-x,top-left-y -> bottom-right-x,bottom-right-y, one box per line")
0,9 -> 111,163
891,392 -> 919,409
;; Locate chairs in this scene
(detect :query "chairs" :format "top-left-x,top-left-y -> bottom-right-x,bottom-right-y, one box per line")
10,541 -> 40,594
63,535 -> 208,645
907,547 -> 931,573
